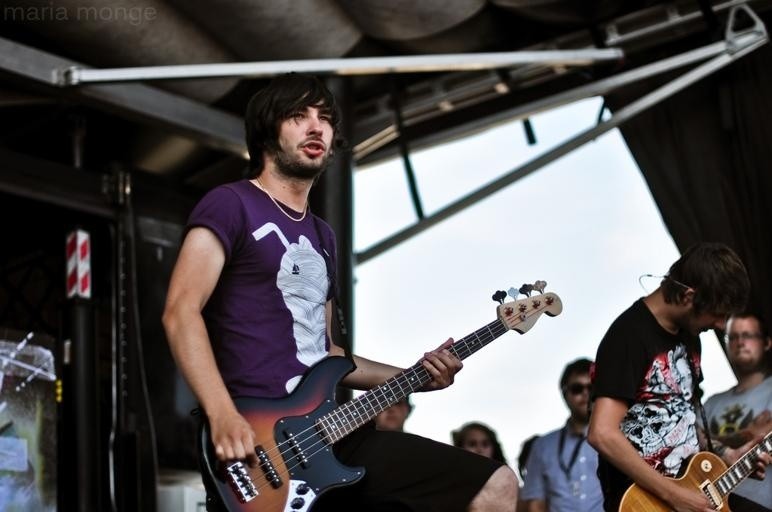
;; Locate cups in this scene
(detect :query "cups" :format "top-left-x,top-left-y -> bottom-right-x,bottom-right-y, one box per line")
564,382 -> 592,395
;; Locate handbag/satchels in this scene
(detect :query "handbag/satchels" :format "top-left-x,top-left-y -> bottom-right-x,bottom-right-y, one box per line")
706,438 -> 727,457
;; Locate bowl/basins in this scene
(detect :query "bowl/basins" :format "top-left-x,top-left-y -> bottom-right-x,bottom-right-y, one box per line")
256,176 -> 309,222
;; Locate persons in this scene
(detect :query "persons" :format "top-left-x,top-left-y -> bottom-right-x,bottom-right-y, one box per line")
516,436 -> 541,511
366,392 -> 415,433
450,420 -> 506,463
697,310 -> 772,511
160,67 -> 522,511
583,236 -> 772,512
521,359 -> 606,511
